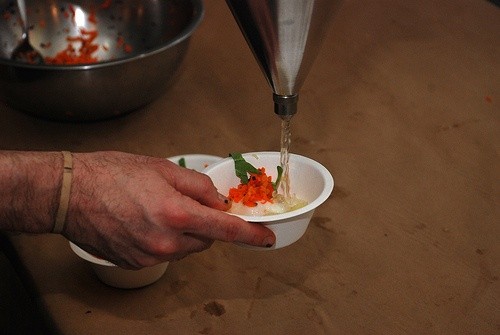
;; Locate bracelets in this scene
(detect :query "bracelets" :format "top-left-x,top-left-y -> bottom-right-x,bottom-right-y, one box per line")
51,150 -> 74,234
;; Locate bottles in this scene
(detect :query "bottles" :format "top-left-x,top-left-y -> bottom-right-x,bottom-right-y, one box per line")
226,1 -> 342,120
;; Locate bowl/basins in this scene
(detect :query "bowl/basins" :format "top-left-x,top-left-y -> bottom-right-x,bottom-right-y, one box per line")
0,0 -> 207,121
204,150 -> 335,252
165,152 -> 225,174
69,243 -> 170,290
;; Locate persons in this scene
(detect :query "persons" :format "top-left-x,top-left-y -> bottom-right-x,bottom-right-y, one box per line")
0,148 -> 275,272
